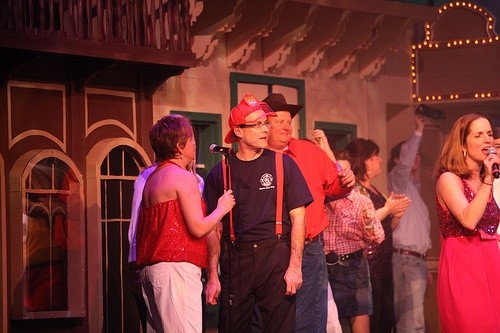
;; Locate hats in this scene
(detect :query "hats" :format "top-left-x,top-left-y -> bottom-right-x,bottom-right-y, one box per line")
224,94 -> 279,144
262,93 -> 304,120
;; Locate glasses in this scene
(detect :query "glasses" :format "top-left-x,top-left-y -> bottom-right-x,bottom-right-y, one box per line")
238,121 -> 271,129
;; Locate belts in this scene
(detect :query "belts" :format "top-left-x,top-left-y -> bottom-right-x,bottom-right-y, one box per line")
392,248 -> 425,259
325,249 -> 363,266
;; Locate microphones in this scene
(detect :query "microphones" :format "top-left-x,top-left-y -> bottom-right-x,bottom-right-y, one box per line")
487,148 -> 500,179
209,144 -> 238,154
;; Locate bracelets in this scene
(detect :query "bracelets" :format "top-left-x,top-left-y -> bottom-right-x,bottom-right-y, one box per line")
482,180 -> 494,186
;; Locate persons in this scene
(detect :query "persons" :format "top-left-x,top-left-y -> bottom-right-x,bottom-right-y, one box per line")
133,114 -> 237,333
436,113 -> 500,333
490,126 -> 500,209
387,112 -> 432,333
129,92 -> 412,333
